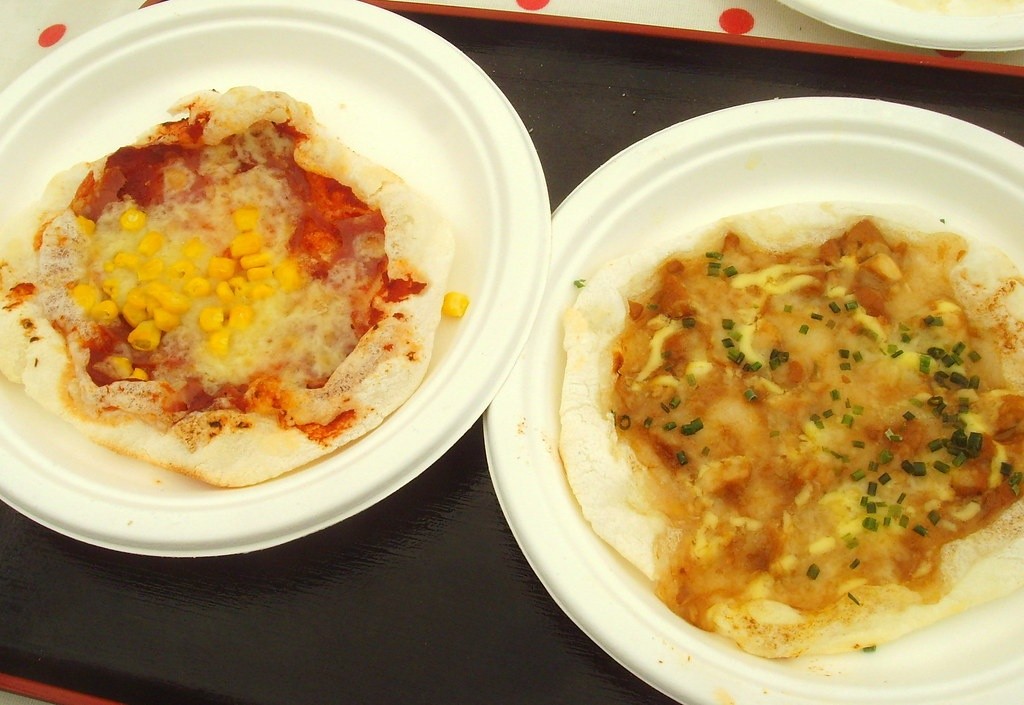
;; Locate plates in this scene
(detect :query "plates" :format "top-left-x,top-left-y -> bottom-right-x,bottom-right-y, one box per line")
1,2 -> 550,558
774,0 -> 1024,53
480,92 -> 1024,705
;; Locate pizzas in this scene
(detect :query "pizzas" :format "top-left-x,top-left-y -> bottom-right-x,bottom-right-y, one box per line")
0,84 -> 457,484
559,203 -> 1024,659
